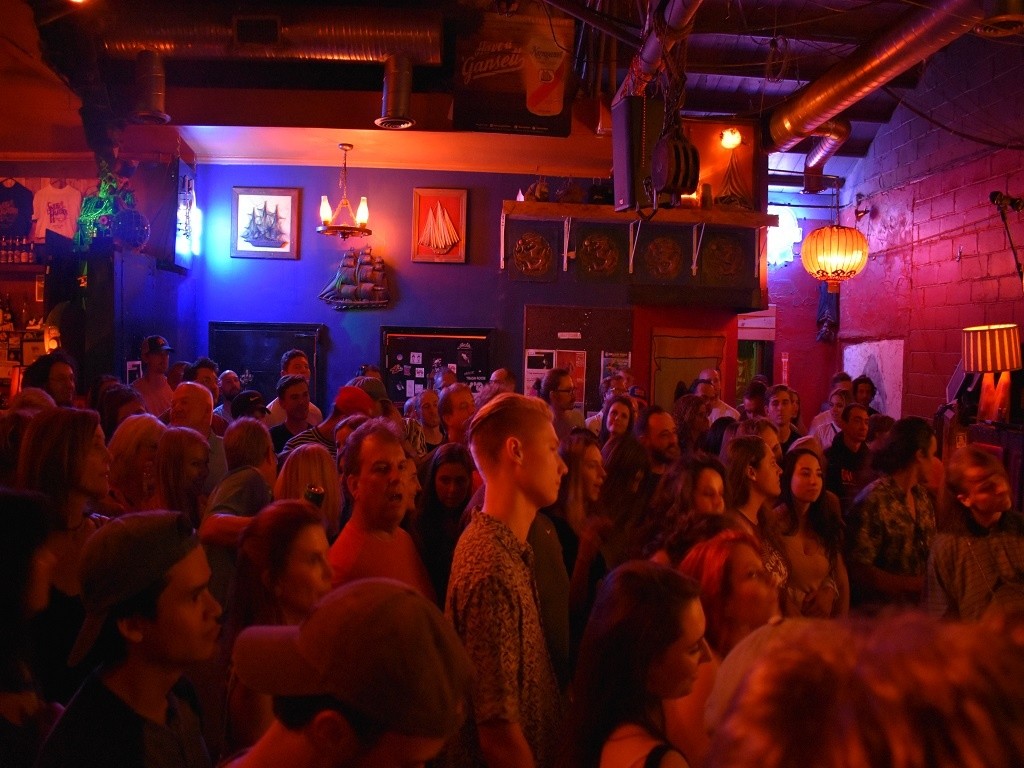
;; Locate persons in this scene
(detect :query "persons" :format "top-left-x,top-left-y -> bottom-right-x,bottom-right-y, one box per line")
0,328 -> 1024,768
860,353 -> 890,415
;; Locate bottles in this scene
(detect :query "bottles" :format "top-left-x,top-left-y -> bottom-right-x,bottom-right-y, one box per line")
4,301 -> 17,329
0,234 -> 37,264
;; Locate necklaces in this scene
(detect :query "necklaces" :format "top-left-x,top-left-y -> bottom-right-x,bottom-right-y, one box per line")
118,671 -> 166,725
606,727 -> 646,751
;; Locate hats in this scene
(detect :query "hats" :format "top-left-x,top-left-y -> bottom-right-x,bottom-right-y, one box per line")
232,579 -> 475,737
143,335 -> 173,356
231,391 -> 272,418
68,510 -> 198,667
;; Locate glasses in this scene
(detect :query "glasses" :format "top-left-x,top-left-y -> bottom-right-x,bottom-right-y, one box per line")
555,387 -> 577,395
272,451 -> 279,466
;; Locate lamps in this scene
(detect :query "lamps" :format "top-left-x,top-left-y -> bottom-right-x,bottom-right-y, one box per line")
315,143 -> 373,237
963,321 -> 1023,424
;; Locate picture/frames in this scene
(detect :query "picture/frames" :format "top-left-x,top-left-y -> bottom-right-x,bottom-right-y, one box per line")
379,326 -> 494,420
410,188 -> 466,263
210,322 -> 326,418
231,186 -> 301,259
521,302 -> 636,425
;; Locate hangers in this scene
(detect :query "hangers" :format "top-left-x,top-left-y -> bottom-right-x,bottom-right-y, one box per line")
1,168 -> 16,184
52,168 -> 69,186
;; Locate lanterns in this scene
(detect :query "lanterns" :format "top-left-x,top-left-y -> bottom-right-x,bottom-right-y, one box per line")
801,224 -> 869,293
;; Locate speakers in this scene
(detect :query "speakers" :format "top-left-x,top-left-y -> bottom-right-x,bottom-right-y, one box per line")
611,96 -> 673,211
